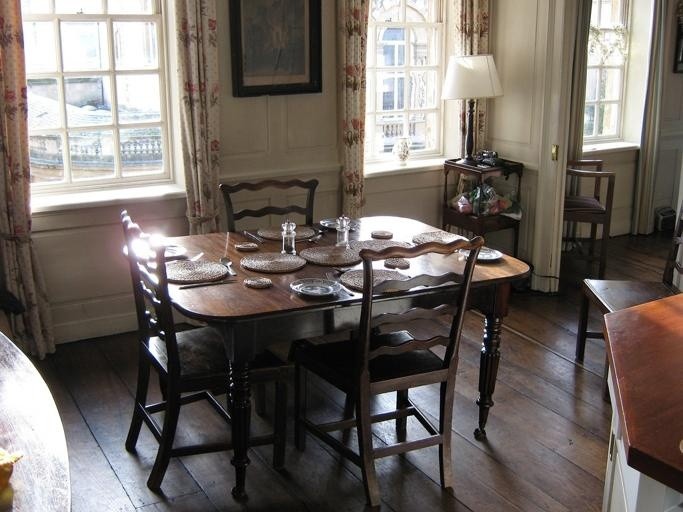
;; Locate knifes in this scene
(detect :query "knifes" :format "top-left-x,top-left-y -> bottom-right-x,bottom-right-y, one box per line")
179,279 -> 236,290
242,230 -> 264,244
190,252 -> 204,261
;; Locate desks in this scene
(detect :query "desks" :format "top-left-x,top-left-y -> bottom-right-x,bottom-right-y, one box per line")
600,292 -> 683,511
1,329 -> 72,512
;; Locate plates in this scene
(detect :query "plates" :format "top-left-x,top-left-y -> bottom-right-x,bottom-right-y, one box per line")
159,243 -> 186,258
320,217 -> 357,230
458,246 -> 503,261
289,277 -> 343,297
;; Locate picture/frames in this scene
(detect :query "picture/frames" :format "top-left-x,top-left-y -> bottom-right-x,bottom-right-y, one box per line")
673,1 -> 682,73
228,0 -> 323,99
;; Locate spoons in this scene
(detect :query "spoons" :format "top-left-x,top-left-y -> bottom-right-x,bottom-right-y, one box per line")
219,257 -> 237,276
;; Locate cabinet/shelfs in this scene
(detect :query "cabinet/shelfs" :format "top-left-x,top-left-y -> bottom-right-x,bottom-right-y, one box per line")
443,156 -> 523,260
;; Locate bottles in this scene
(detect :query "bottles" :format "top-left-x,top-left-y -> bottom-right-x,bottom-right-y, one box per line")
280,218 -> 296,257
335,214 -> 351,247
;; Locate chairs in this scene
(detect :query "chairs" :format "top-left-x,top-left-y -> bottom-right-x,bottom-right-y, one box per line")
119,209 -> 295,493
563,160 -> 616,278
219,179 -> 335,415
285,235 -> 486,507
574,199 -> 682,400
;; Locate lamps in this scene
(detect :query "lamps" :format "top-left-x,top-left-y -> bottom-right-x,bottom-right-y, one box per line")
441,54 -> 503,165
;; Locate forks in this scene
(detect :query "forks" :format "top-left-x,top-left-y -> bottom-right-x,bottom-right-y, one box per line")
326,271 -> 353,297
